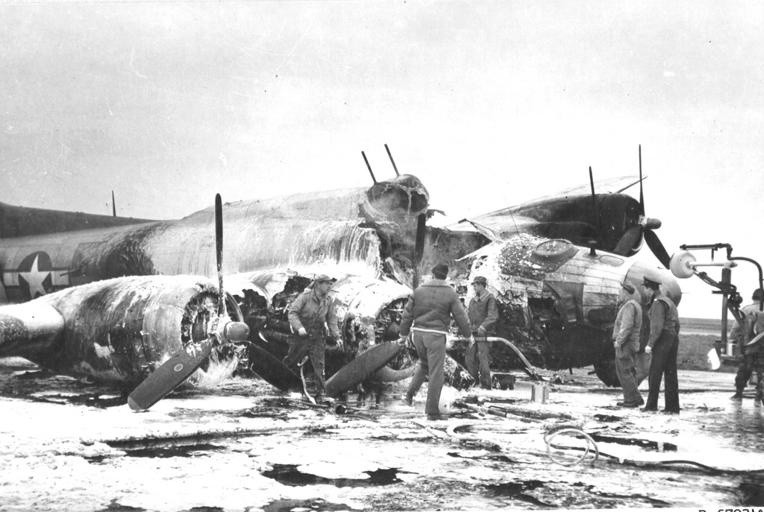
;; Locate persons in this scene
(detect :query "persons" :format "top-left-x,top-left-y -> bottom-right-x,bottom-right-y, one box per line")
639,276 -> 680,414
282,274 -> 343,385
463,276 -> 499,389
610,284 -> 646,408
398,265 -> 474,420
729,289 -> 764,400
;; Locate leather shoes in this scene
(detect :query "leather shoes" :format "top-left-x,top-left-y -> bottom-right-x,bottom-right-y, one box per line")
615,399 -> 645,407
639,407 -> 680,414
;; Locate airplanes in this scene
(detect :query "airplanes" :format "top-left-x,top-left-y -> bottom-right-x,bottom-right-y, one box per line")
0,135 -> 744,420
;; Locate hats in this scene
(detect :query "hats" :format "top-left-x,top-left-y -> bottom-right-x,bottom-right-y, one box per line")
640,275 -> 662,287
619,281 -> 635,295
470,275 -> 488,287
430,261 -> 449,275
313,272 -> 338,284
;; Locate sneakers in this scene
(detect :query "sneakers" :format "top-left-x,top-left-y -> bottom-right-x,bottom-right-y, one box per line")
730,395 -> 762,408
314,394 -> 330,405
400,396 -> 415,407
426,414 -> 448,421
